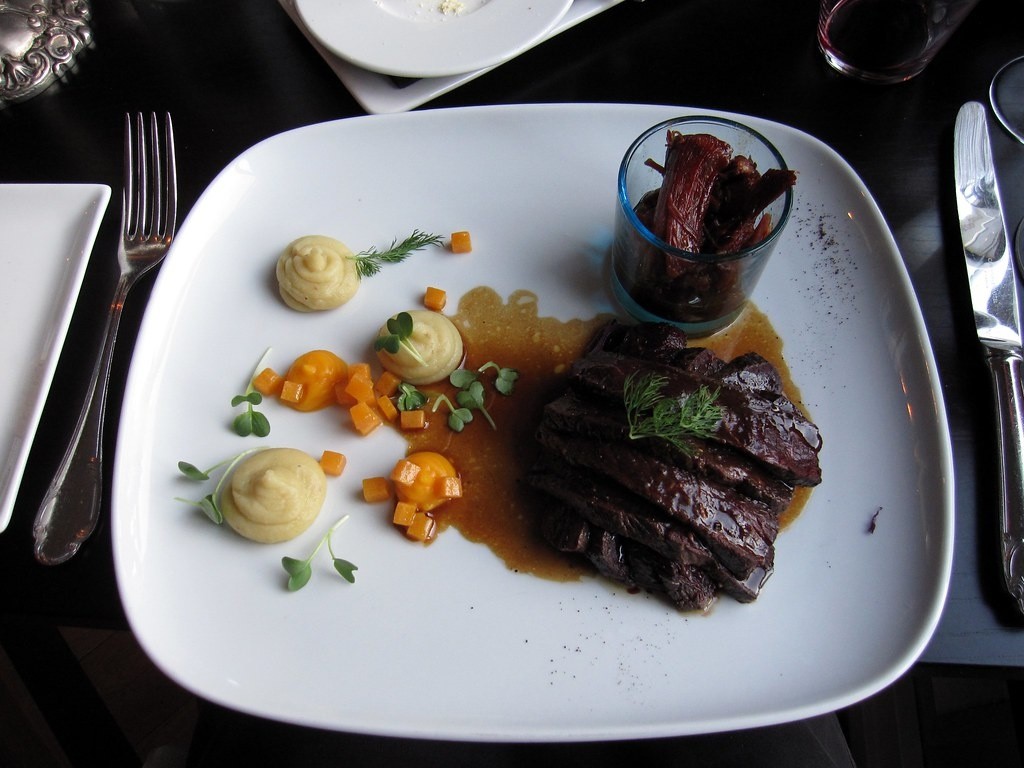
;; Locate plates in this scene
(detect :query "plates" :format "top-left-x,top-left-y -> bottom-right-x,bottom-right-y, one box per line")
294,0 -> 574,77
0,184 -> 112,535
112,103 -> 957,742
283,0 -> 625,112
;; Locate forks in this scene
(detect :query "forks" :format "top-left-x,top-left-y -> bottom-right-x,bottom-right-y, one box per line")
35,111 -> 177,569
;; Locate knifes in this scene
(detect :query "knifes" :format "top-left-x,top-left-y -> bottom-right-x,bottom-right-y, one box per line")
954,99 -> 1024,617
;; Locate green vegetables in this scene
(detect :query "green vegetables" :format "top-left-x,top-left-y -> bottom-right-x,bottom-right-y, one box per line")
174,230 -> 519,592
624,368 -> 725,458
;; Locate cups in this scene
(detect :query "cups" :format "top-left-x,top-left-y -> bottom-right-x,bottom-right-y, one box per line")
818,0 -> 977,82
609,116 -> 793,339
